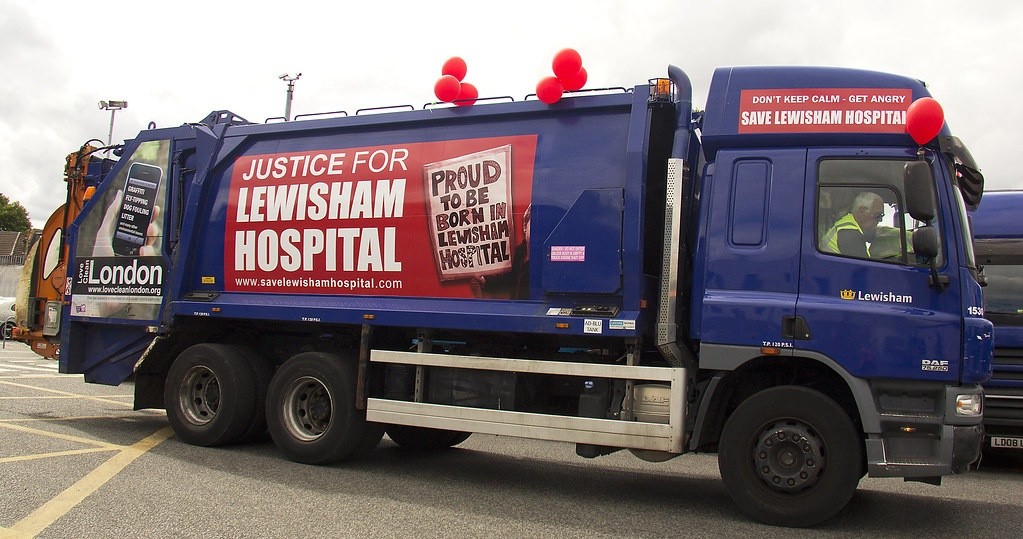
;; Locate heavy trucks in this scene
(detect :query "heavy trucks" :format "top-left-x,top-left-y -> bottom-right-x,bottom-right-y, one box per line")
11,61 -> 994,526
893,188 -> 1023,469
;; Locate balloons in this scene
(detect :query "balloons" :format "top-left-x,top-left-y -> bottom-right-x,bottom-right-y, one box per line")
435,57 -> 478,107
537,48 -> 587,104
905,96 -> 945,145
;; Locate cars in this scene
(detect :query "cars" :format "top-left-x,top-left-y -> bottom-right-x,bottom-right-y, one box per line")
0,296 -> 16,339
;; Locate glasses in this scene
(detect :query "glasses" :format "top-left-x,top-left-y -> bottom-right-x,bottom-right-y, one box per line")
864,206 -> 885,221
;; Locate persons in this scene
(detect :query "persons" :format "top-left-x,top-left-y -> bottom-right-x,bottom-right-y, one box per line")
819,191 -> 883,260
92,189 -> 160,257
